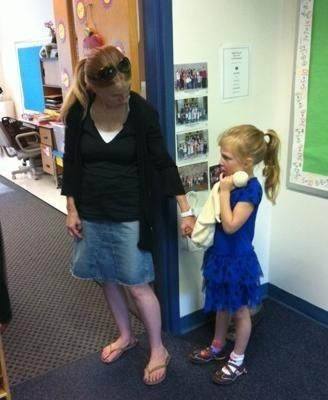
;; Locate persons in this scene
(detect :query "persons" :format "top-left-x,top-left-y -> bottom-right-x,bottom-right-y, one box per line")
56,47 -> 198,387
175,65 -> 221,189
183,125 -> 281,387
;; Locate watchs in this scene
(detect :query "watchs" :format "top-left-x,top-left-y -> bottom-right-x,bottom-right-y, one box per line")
181,208 -> 195,218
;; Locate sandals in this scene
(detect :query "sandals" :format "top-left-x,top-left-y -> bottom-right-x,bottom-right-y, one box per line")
211,362 -> 247,387
189,343 -> 227,364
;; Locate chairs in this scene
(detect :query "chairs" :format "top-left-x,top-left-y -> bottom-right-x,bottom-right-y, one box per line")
0,117 -> 42,181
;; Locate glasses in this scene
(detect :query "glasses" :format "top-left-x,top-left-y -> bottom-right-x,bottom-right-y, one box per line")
96,55 -> 132,83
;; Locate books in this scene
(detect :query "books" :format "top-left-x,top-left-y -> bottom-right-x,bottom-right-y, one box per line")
45,94 -> 61,113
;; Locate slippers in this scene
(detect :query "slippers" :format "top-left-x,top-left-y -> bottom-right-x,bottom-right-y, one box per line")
100,336 -> 138,365
142,351 -> 171,386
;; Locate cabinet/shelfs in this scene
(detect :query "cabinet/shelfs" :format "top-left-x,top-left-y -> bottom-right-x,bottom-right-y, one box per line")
21,0 -> 139,188
